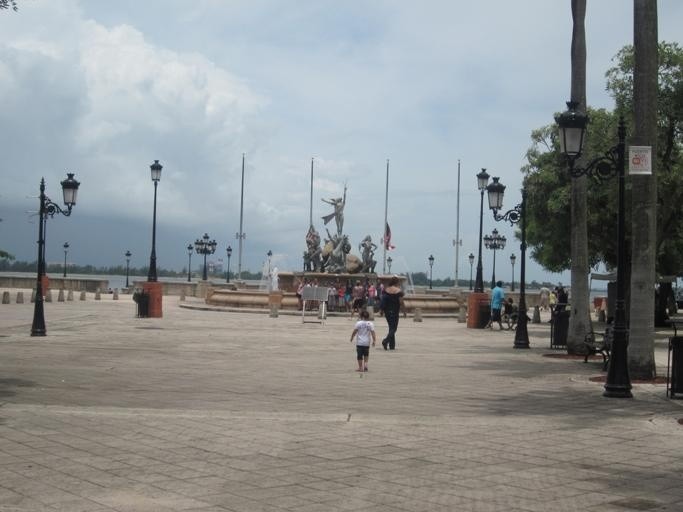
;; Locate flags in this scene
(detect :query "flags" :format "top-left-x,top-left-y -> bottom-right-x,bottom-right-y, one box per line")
385,223 -> 395,250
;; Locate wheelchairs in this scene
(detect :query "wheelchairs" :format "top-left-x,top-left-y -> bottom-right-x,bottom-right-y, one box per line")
495,299 -> 522,333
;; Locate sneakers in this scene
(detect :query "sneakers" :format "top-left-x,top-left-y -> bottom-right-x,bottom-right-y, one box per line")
382,340 -> 387,350
355,366 -> 368,372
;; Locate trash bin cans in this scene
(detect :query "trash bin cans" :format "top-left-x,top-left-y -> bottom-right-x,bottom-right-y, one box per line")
133,287 -> 150,318
549,303 -> 571,350
478,298 -> 492,329
665,319 -> 683,399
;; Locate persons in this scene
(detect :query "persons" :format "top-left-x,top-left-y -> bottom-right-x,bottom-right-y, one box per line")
506,297 -> 531,321
382,277 -> 406,350
485,281 -> 508,330
351,311 -> 376,371
539,282 -> 568,323
296,277 -> 386,319
303,197 -> 377,273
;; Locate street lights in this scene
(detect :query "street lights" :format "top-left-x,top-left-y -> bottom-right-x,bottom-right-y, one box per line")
25,169 -> 81,335
465,251 -> 474,290
185,242 -> 194,282
385,255 -> 393,275
509,253 -> 516,292
427,254 -> 436,290
60,239 -> 70,277
481,228 -> 507,290
484,180 -> 532,347
192,232 -> 217,281
122,248 -> 132,288
266,249 -> 271,276
553,92 -> 643,403
224,246 -> 232,283
146,159 -> 163,283
472,159 -> 491,293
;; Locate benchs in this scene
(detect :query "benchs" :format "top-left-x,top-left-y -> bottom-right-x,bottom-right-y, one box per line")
584,327 -> 611,372
